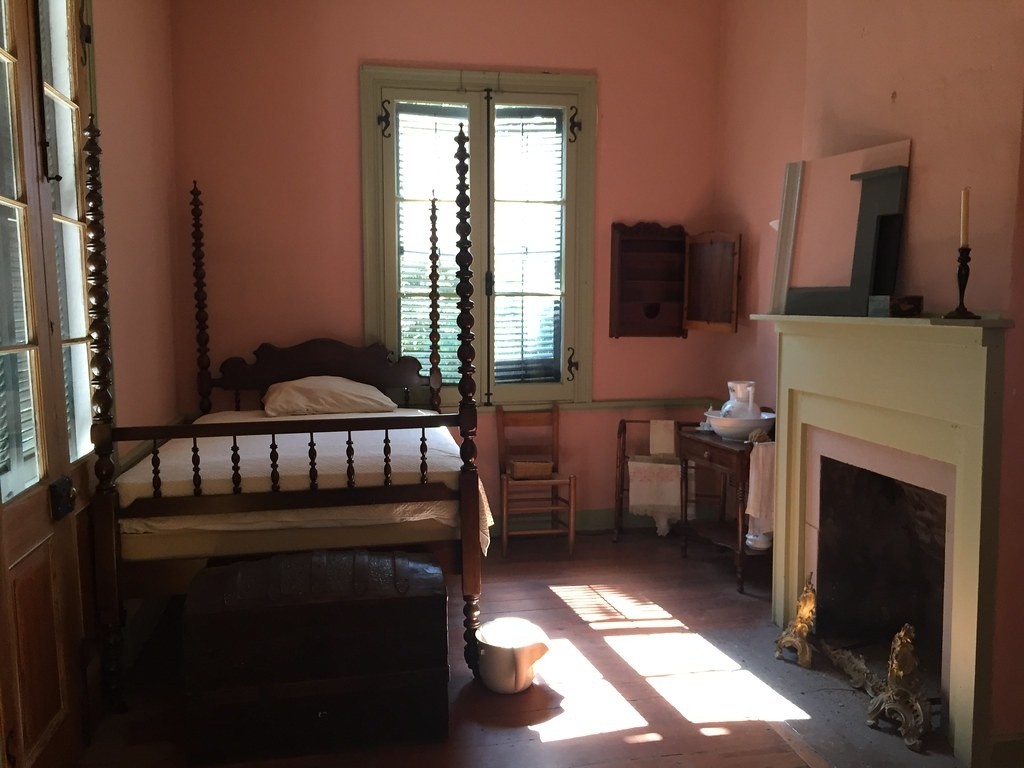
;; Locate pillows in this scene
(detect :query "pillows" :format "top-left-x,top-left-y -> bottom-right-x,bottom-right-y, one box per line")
260,375 -> 399,417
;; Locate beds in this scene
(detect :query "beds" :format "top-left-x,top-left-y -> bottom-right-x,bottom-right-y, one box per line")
92,338 -> 483,688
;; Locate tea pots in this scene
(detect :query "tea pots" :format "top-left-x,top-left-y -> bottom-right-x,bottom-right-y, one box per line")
720,380 -> 762,420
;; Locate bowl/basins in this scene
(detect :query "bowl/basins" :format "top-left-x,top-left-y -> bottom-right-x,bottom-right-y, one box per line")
705,409 -> 776,442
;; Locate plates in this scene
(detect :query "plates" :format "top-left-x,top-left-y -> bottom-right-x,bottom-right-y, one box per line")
696,427 -> 713,434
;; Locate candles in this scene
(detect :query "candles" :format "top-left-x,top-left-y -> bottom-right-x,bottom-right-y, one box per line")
959,185 -> 973,249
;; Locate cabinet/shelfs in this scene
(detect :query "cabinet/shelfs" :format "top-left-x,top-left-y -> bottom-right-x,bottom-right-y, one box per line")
611,221 -> 742,341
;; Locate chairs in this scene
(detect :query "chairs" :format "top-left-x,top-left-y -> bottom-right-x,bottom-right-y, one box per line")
495,403 -> 575,560
